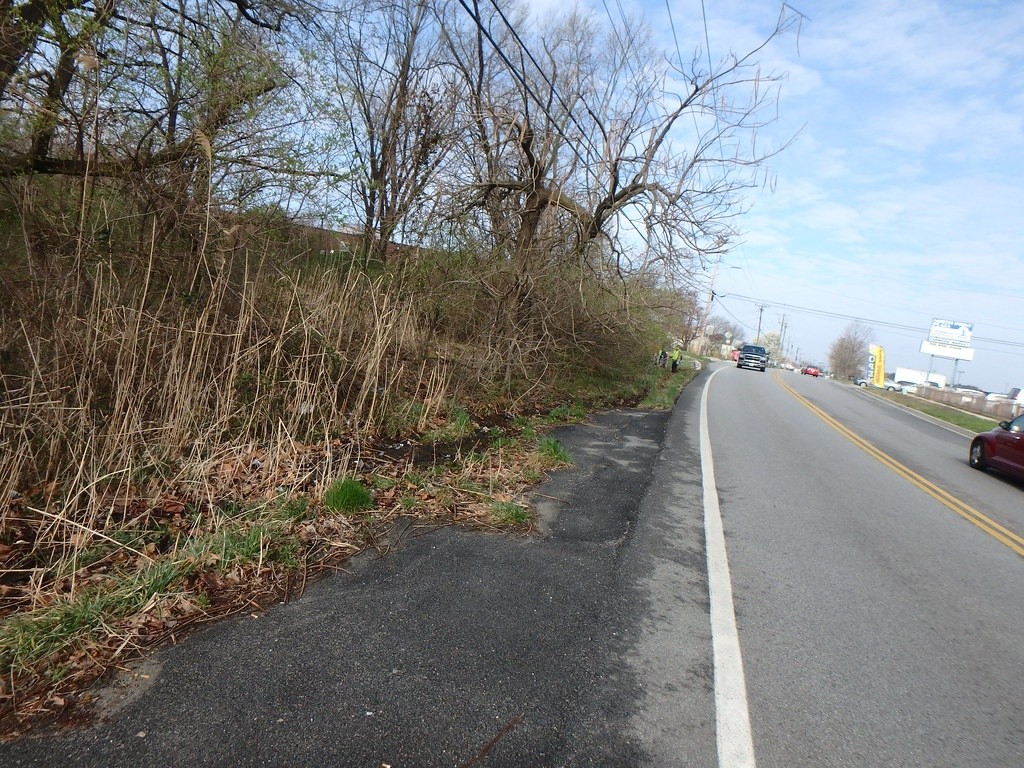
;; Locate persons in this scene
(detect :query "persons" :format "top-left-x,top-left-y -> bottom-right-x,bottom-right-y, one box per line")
669,344 -> 683,372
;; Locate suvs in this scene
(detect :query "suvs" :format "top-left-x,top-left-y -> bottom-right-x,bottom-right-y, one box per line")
736,345 -> 770,372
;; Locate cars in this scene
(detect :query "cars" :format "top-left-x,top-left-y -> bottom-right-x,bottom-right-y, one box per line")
853,376 -> 903,393
800,365 -> 819,377
969,414 -> 1024,484
818,371 -> 824,377
896,381 -> 918,393
